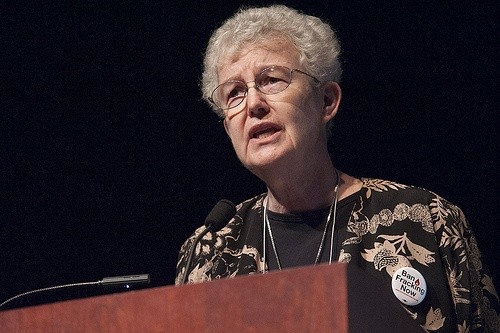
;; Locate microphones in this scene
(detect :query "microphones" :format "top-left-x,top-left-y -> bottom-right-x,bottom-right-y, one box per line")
182,199 -> 237,284
0,274 -> 151,309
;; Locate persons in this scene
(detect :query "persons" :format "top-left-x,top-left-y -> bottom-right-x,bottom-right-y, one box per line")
174,5 -> 500,333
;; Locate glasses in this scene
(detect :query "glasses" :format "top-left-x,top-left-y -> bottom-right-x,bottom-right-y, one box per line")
209,63 -> 323,112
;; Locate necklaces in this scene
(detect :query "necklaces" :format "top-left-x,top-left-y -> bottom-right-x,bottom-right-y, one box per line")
263,168 -> 341,275
